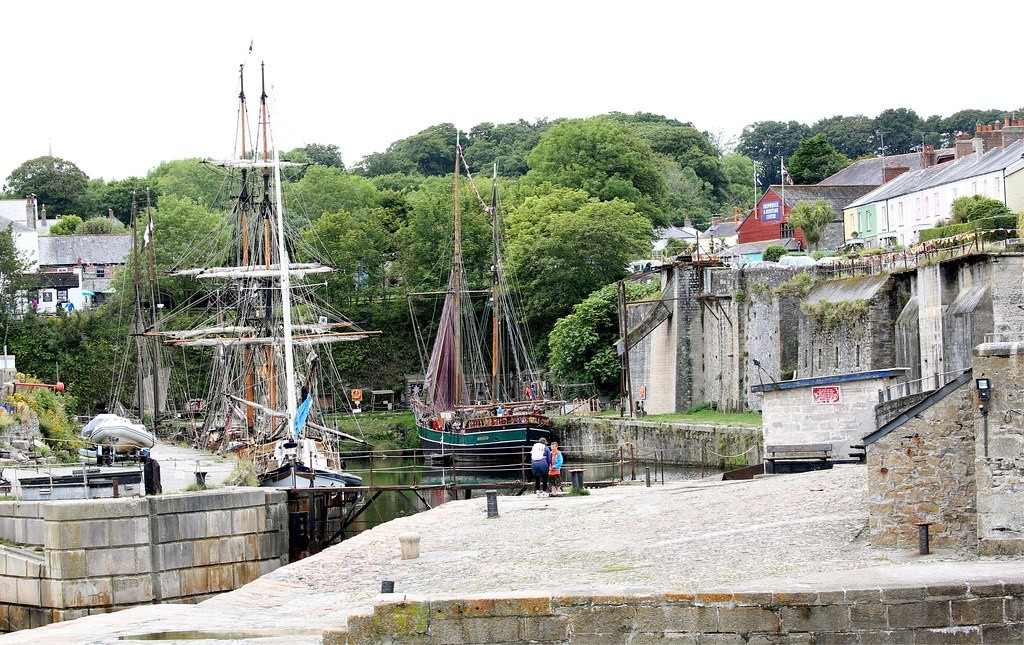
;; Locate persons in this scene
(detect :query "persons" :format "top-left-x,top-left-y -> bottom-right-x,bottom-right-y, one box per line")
96,446 -> 113,467
56,299 -> 74,317
429,418 -> 463,432
530,438 -> 563,498
32,298 -> 37,314
490,404 -> 514,416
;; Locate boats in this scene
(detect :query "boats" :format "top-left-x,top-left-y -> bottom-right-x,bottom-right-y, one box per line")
79,456 -> 97,465
78,448 -> 97,456
83,414 -> 157,455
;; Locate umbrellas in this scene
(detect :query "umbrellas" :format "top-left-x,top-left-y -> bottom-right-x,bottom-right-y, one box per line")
82,290 -> 95,296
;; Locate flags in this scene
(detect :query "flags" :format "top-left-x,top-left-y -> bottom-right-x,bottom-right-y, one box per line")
783,166 -> 794,185
294,395 -> 312,437
756,172 -> 762,187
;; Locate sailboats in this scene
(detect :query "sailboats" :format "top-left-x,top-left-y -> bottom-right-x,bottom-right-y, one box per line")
404,123 -> 569,467
98,58 -> 385,548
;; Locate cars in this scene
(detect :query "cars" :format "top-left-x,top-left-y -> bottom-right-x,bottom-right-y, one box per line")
751,261 -> 777,268
779,255 -> 816,269
